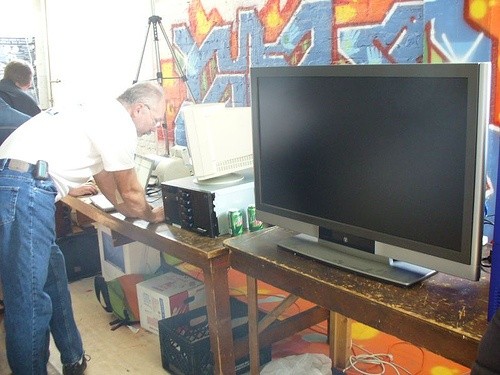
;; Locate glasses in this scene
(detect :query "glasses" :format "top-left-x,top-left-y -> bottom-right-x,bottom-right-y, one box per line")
144,104 -> 161,128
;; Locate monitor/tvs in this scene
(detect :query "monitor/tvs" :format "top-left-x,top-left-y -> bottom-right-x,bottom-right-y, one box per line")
250,64 -> 485,289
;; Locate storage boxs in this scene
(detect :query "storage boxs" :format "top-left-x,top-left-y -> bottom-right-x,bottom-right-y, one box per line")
136,271 -> 206,337
95,222 -> 161,325
157,296 -> 271,375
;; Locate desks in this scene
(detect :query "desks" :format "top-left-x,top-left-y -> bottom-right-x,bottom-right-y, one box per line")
223,228 -> 492,375
58,180 -> 331,374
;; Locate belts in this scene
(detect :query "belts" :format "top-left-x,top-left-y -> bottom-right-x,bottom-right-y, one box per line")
1,159 -> 34,173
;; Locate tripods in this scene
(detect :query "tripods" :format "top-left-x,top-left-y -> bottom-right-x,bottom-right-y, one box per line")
131,0 -> 198,156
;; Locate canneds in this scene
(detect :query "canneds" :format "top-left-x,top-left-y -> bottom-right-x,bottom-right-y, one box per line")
227,209 -> 244,237
247,205 -> 264,232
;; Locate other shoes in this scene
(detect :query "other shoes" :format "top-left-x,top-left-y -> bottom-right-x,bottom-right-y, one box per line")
63,350 -> 91,375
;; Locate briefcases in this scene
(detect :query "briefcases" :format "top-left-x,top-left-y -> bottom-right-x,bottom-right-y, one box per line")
57,232 -> 101,281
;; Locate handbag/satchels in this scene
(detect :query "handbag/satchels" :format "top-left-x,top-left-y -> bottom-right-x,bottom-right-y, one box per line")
95,262 -> 154,321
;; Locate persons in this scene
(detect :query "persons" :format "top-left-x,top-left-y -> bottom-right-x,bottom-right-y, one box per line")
0,81 -> 166,375
0,60 -> 41,145
